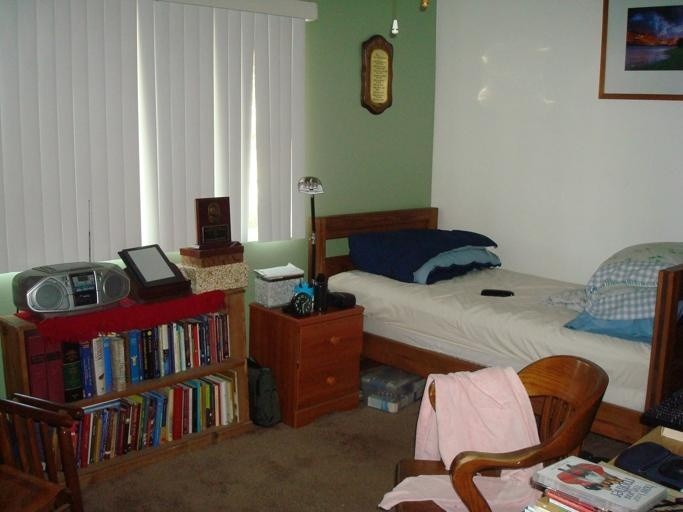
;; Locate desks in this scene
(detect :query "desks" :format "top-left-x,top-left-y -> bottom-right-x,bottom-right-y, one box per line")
522,426 -> 683,512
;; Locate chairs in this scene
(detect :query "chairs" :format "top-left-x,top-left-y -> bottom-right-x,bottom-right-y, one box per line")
0,391 -> 84,512
395,355 -> 607,512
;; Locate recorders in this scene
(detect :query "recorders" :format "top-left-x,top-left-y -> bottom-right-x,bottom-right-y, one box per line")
12,261 -> 130,311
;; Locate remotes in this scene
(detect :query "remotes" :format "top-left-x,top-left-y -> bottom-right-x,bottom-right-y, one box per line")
480,288 -> 515,297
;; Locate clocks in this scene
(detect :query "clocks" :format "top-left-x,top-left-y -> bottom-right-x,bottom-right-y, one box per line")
289,291 -> 313,318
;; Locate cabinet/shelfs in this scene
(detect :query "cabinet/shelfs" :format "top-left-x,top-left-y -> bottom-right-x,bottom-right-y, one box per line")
0,287 -> 255,491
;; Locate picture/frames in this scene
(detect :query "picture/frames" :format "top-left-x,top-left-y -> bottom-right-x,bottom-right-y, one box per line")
361,35 -> 392,115
598,0 -> 683,101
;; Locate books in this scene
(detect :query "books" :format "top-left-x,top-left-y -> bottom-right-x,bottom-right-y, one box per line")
22,313 -> 239,472
252,262 -> 305,281
521,454 -> 667,512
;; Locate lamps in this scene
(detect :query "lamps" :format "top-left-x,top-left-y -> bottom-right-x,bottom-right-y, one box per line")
389,0 -> 428,35
298,176 -> 324,286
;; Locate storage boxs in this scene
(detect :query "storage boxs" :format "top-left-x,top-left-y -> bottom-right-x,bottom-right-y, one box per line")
177,264 -> 249,295
254,277 -> 303,308
178,244 -> 244,268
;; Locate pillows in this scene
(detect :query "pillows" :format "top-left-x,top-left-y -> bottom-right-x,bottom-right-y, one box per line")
539,241 -> 683,305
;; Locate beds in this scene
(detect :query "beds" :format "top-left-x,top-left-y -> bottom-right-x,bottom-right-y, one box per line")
308,208 -> 683,447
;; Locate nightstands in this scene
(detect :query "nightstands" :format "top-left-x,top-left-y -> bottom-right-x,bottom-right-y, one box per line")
250,301 -> 364,428
